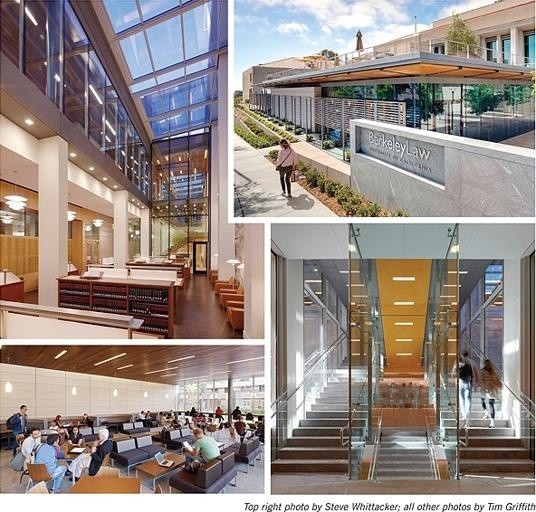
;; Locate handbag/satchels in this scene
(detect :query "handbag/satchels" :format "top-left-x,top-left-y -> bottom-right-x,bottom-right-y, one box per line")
184,460 -> 204,472
276,166 -> 281,170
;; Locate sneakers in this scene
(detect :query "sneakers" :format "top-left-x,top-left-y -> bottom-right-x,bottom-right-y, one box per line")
282,191 -> 291,197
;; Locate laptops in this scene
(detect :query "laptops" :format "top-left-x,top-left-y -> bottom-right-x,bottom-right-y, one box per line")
155,452 -> 175,468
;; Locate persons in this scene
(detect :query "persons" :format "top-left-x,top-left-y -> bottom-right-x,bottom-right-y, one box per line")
275,138 -> 298,198
451,350 -> 480,428
6,403 -> 264,492
476,358 -> 504,428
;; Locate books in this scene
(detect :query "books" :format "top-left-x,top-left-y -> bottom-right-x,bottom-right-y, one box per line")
59,281 -> 169,336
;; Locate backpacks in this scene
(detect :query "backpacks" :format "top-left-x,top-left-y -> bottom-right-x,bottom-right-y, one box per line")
7,414 -> 19,430
23,443 -> 45,475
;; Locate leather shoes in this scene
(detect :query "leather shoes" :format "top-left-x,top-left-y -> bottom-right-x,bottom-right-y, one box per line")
65,470 -> 70,476
69,477 -> 78,481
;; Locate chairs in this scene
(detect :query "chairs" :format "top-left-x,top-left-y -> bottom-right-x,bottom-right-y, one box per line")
1,410 -> 265,494
2,250 -> 247,337
